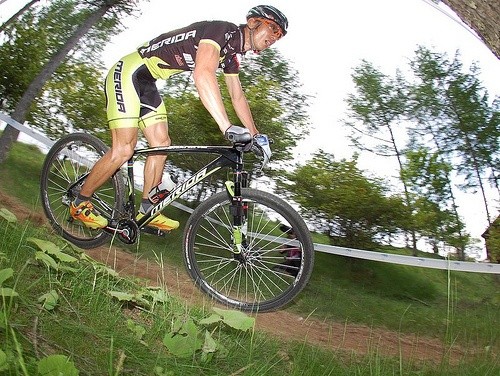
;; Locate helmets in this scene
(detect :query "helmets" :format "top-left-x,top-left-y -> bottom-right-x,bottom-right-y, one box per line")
246,5 -> 288,37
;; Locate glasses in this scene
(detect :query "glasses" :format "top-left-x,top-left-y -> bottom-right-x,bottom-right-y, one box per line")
255,17 -> 284,40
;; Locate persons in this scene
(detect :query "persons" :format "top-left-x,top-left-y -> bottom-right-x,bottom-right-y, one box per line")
69,4 -> 288,230
280,228 -> 302,279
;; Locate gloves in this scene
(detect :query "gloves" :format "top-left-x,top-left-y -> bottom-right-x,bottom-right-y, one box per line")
224,125 -> 253,152
252,145 -> 261,154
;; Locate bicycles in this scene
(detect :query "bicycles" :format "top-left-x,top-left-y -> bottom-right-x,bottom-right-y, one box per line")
39,131 -> 316,314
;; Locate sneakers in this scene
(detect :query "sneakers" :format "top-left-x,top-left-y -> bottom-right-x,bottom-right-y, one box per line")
135,210 -> 180,231
70,200 -> 108,230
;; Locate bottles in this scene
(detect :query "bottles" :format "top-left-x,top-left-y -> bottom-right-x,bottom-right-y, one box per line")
148,175 -> 179,204
